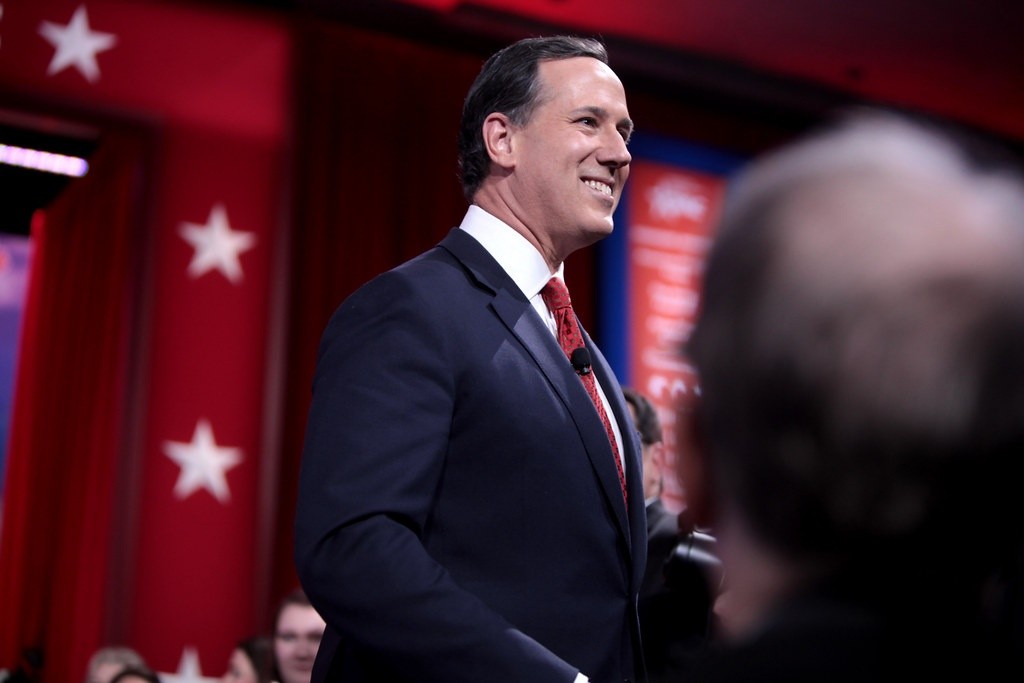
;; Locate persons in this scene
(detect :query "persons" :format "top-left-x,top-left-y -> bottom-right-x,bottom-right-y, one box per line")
295,34 -> 647,683
111,666 -> 159,683
222,638 -> 270,683
622,388 -> 674,683
681,119 -> 1024,683
267,594 -> 327,683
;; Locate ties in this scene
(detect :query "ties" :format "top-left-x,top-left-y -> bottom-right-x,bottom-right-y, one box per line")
540,277 -> 629,516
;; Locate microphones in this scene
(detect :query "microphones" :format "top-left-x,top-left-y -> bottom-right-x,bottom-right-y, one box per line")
571,346 -> 592,376
